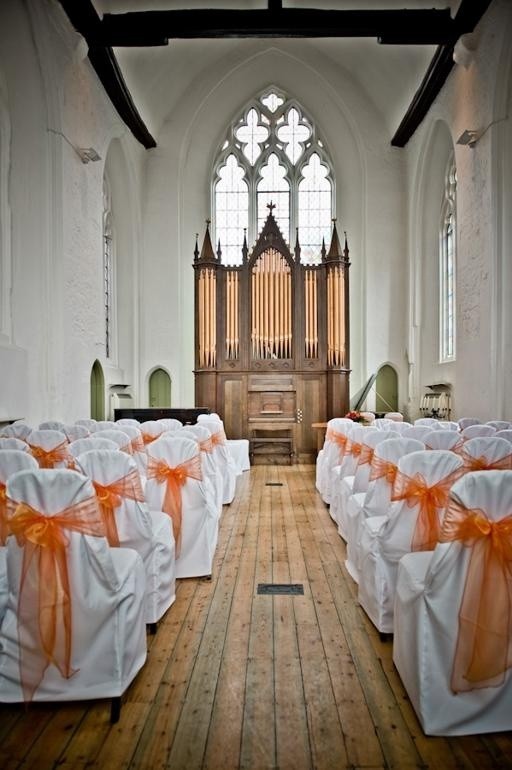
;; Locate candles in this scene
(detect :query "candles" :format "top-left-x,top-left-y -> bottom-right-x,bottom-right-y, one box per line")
420,392 -> 451,409
